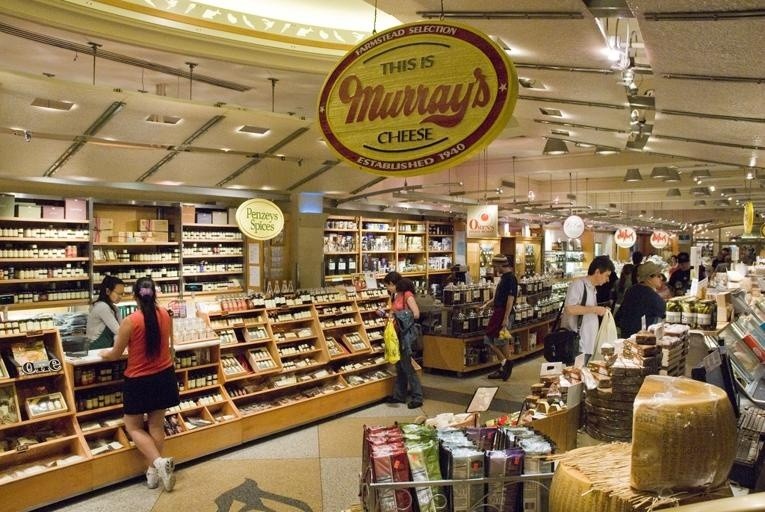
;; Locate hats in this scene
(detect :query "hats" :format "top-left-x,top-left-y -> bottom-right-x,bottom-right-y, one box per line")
492,254 -> 508,266
638,261 -> 660,282
678,252 -> 689,263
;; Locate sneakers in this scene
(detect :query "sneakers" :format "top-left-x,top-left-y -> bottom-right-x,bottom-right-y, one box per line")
488,359 -> 512,380
146,458 -> 176,492
387,397 -> 423,408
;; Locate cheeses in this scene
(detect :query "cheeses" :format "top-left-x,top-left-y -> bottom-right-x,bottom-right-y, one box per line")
549,376 -> 738,512
526,367 -> 581,416
584,328 -> 663,443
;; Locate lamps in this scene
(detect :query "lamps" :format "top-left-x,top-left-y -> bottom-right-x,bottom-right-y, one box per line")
605,16 -> 656,150
542,138 -> 570,156
594,146 -> 620,156
623,166 -> 744,215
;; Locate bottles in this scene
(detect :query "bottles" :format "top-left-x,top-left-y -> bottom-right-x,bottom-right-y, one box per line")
14,281 -> 90,304
118,249 -> 180,262
0,262 -> 89,279
94,265 -> 179,280
75,354 -> 224,412
265,280 -> 295,298
0,316 -> 54,335
0,243 -> 82,258
277,343 -> 312,370
183,259 -> 243,272
172,317 -> 214,341
297,287 -> 380,303
0,225 -> 89,239
183,231 -> 242,240
429,224 -> 449,235
184,244 -> 242,255
221,293 -> 265,312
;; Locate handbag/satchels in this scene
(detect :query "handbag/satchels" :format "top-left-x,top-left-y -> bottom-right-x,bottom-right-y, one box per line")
384,321 -> 401,364
544,328 -> 580,366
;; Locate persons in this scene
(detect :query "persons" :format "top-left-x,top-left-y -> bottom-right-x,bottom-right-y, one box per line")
375,271 -> 425,409
446,264 -> 471,286
86,275 -> 125,349
471,254 -> 518,381
99,278 -> 180,492
597,242 -> 759,339
559,256 -> 616,369
